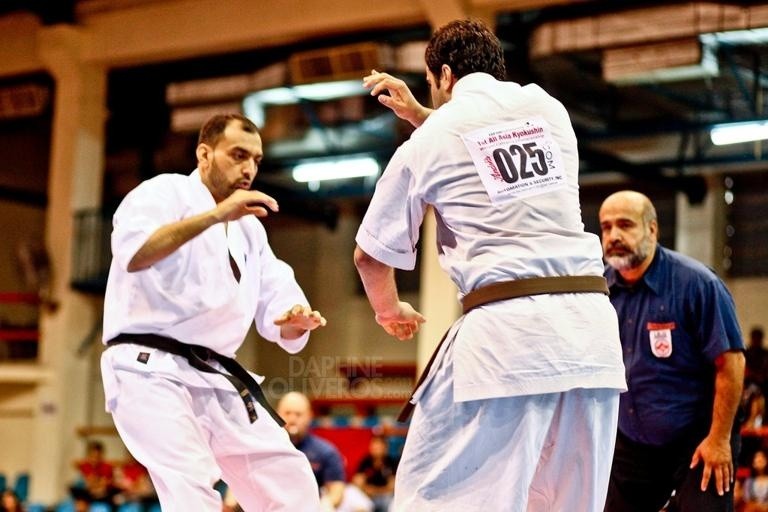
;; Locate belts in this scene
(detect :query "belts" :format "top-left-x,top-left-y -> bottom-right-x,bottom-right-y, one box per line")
459,275 -> 612,314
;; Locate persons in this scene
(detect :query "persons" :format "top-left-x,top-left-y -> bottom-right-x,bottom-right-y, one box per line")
600,189 -> 746,512
353,19 -> 629,511
99,115 -> 327,512
